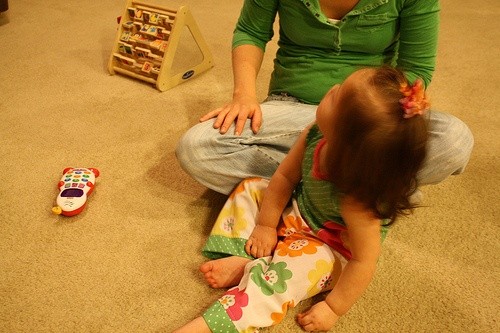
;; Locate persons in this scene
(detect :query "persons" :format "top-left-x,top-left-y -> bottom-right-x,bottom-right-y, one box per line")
174,0 -> 474,197
175,67 -> 430,333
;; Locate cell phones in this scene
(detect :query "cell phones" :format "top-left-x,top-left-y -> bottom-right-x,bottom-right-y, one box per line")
51,167 -> 99,217
